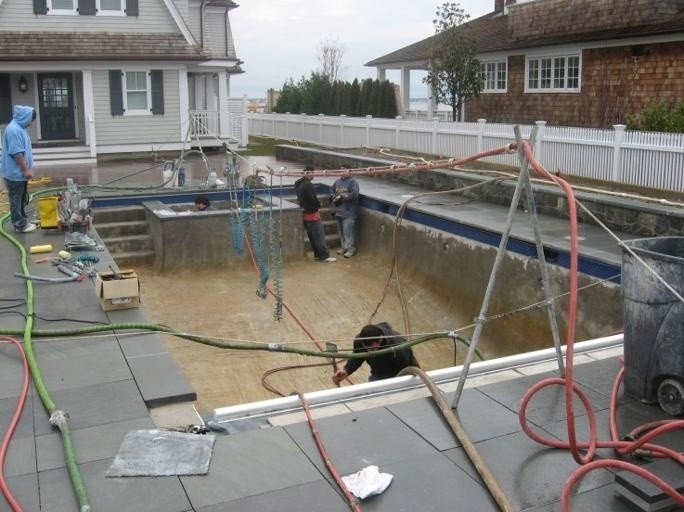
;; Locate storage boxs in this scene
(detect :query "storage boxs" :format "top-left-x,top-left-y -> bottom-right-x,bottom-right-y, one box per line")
96,268 -> 141,311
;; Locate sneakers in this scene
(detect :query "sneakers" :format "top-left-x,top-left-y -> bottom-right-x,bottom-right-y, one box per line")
314,257 -> 337,262
15,223 -> 36,233
336,247 -> 357,258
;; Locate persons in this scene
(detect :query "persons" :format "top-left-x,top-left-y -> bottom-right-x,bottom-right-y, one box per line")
332,321 -> 422,387
329,164 -> 360,258
293,167 -> 337,262
186,195 -> 216,212
0,105 -> 40,233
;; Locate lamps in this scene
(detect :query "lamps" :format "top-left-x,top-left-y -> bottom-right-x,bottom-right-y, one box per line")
19,76 -> 27,93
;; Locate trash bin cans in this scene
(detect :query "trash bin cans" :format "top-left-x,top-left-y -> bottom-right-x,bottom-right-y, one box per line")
618,236 -> 684,416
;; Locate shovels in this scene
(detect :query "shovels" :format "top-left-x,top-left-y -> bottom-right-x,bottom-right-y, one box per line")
326,343 -> 340,388
